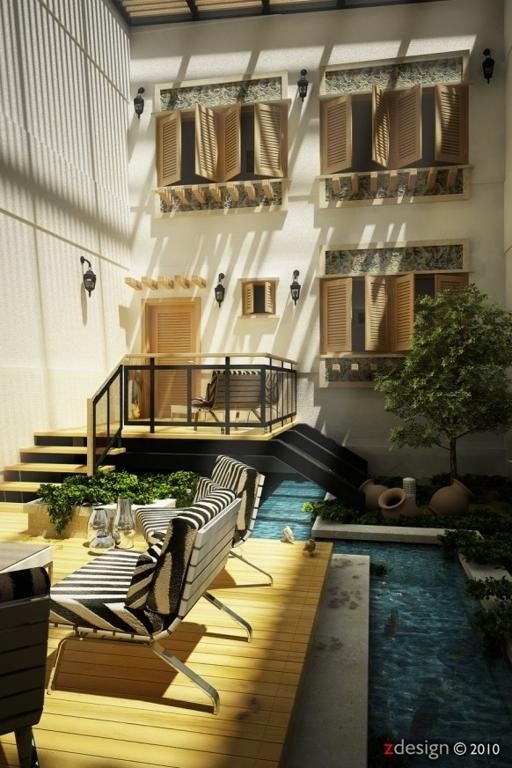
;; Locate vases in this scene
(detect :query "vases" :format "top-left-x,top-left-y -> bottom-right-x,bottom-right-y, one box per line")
375,483 -> 418,521
357,477 -> 390,511
427,477 -> 471,519
112,493 -> 136,548
87,506 -> 114,547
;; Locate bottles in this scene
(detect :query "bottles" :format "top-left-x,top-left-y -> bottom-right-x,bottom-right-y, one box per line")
86,495 -> 136,554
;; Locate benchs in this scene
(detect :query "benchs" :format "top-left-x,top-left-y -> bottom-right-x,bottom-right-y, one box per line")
186,368 -> 268,433
243,369 -> 287,426
48,487 -> 254,719
134,451 -> 276,591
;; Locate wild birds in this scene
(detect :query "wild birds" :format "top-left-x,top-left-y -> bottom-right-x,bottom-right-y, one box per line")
281,525 -> 296,546
302,539 -> 317,558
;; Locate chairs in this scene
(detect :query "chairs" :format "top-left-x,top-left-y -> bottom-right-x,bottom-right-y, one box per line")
1,560 -> 55,767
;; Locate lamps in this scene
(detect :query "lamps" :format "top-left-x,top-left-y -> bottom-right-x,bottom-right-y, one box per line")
132,86 -> 152,120
479,45 -> 497,86
295,68 -> 316,103
290,269 -> 302,307
211,272 -> 228,310
79,255 -> 97,298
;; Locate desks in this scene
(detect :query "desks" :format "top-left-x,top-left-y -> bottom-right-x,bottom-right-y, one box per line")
169,403 -> 202,422
0,539 -> 54,584
